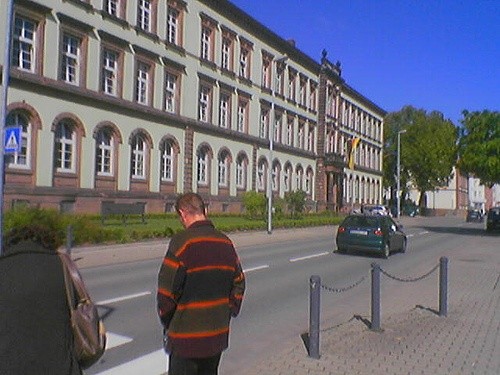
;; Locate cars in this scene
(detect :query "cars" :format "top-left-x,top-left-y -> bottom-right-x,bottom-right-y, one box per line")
337,214 -> 407,257
487,207 -> 500,234
466,207 -> 483,223
349,205 -> 392,217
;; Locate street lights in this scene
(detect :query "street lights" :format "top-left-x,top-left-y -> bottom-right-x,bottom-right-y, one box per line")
398,130 -> 407,223
267,55 -> 289,234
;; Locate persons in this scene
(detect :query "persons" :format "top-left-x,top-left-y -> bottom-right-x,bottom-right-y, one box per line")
0,217 -> 84,375
156,192 -> 246,375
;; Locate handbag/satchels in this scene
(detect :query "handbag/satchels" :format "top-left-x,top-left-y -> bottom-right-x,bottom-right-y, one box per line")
58,252 -> 106,367
